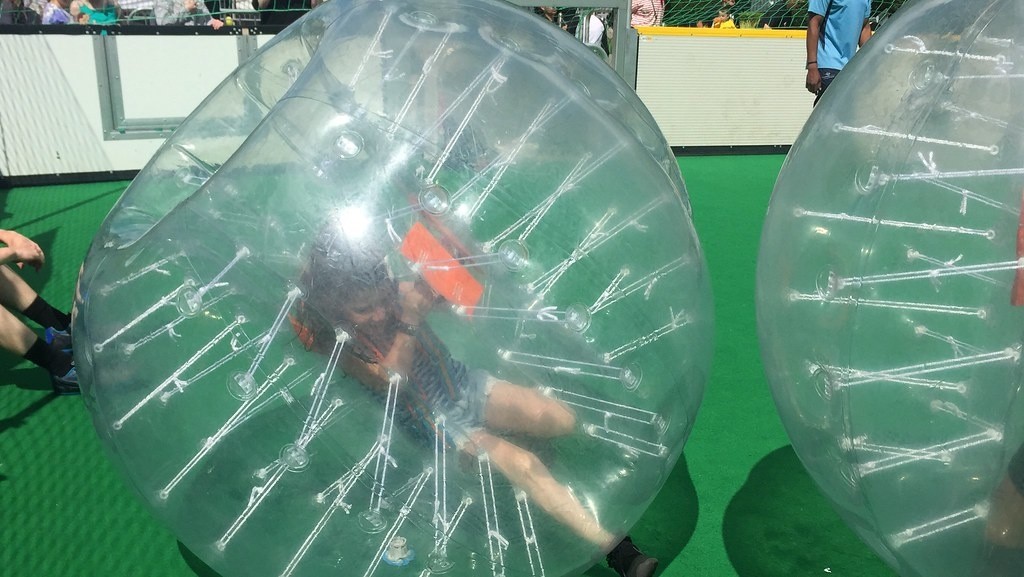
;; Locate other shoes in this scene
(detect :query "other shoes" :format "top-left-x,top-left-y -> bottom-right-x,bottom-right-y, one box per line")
605,535 -> 658,575
49,348 -> 79,394
44,320 -> 71,354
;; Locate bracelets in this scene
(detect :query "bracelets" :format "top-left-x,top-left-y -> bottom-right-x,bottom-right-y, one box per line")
806,61 -> 817,66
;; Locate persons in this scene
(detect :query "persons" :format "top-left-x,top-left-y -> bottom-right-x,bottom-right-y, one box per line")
288,220 -> 660,576
1,0 -> 806,156
0,229 -> 132,394
985,191 -> 1024,551
805,0 -> 874,109
